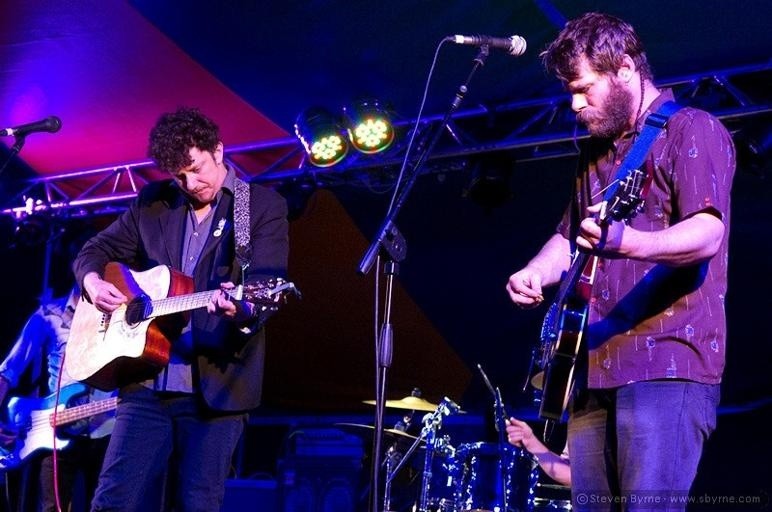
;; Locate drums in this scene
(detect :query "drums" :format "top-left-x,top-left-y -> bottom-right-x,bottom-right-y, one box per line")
417,441 -> 533,511
536,482 -> 572,511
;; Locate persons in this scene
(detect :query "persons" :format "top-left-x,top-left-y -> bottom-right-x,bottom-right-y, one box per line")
503,417 -> 572,487
1,228 -> 117,512
72,106 -> 290,512
503,12 -> 738,512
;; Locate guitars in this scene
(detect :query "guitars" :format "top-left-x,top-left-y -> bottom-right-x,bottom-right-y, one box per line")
0,383 -> 118,472
65,262 -> 302,393
531,169 -> 649,421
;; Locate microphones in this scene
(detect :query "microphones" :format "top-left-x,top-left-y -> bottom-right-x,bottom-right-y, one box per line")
0,115 -> 64,136
447,34 -> 526,56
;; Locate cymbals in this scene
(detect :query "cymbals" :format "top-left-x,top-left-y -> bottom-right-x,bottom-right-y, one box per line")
363,395 -> 467,414
338,423 -> 427,448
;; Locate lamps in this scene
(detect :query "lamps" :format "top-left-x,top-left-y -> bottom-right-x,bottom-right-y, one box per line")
290,95 -> 395,168
